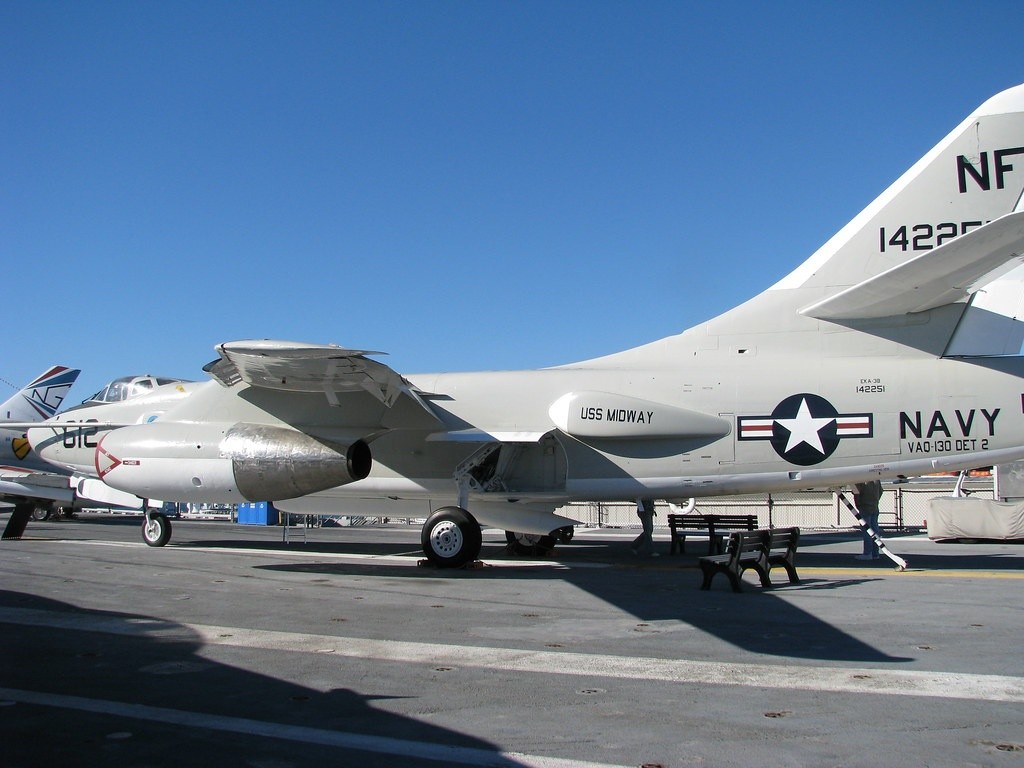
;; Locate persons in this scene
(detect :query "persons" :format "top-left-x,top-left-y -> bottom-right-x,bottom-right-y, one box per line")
850,480 -> 883,559
630,500 -> 661,556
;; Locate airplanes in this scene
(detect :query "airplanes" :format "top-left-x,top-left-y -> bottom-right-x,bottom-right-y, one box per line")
0,83 -> 1024,570
1,365 -> 181,541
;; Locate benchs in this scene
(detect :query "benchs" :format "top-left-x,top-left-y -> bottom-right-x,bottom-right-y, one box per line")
668,514 -> 759,555
698,526 -> 800,592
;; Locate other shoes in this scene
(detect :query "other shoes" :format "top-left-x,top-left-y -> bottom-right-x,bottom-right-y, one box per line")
855,552 -> 880,560
629,546 -> 638,556
